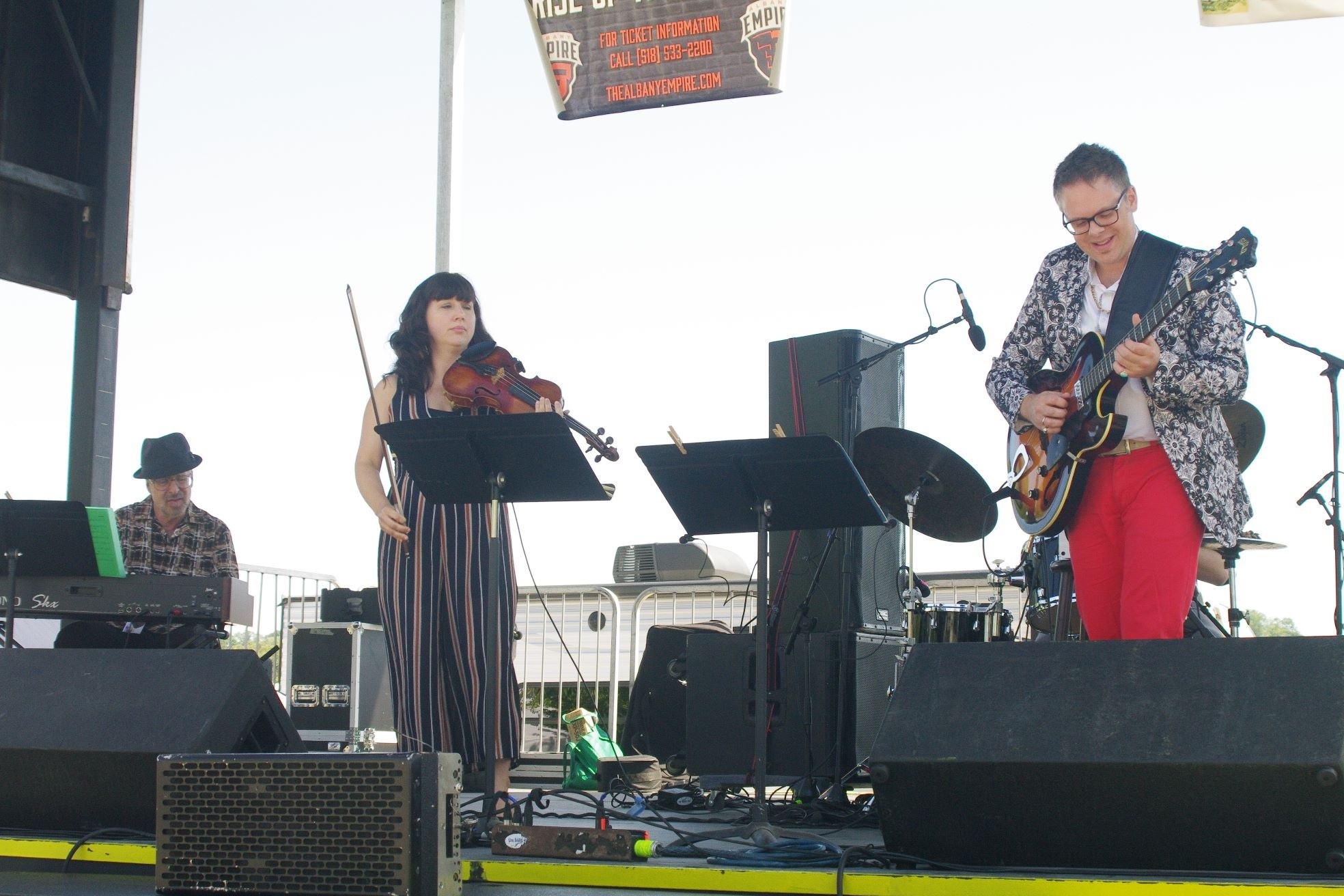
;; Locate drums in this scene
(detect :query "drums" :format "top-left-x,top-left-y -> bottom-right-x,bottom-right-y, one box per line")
1020,536 -> 1087,636
902,603 -> 1013,642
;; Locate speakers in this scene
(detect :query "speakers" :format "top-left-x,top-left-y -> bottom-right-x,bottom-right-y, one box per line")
687,633 -> 905,777
869,634 -> 1344,880
0,647 -> 311,841
769,330 -> 908,636
155,751 -> 464,896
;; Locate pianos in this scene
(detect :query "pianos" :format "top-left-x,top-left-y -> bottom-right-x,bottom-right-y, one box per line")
0,576 -> 254,648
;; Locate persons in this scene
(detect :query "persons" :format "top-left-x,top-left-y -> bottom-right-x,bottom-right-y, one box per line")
55,439 -> 236,649
1191,546 -> 1230,637
986,142 -> 1250,640
357,270 -> 569,838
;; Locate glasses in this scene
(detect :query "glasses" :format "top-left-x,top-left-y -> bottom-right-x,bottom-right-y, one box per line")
149,472 -> 193,490
1062,188 -> 1126,235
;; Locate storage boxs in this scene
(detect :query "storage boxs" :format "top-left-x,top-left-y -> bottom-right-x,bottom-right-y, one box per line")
285,622 -> 396,738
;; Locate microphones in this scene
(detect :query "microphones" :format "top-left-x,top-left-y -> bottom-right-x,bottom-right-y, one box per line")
884,518 -> 897,533
679,535 -> 694,544
956,284 -> 986,351
907,568 -> 931,598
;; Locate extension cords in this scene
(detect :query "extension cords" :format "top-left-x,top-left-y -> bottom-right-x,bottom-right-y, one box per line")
491,825 -> 649,861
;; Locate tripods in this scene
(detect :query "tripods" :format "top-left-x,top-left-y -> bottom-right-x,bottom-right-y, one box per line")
636,434 -> 894,855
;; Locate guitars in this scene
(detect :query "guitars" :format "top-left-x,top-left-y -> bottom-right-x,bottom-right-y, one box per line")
1006,227 -> 1256,537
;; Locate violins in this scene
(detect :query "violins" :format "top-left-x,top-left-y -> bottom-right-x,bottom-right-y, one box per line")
442,340 -> 620,463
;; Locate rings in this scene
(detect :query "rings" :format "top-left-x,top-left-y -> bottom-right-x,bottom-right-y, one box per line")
1120,366 -> 1128,377
1042,417 -> 1047,426
1043,427 -> 1049,434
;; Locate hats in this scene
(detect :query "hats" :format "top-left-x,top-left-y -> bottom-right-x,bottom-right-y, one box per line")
133,432 -> 202,479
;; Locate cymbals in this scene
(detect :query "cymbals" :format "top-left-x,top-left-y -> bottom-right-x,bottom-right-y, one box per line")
854,427 -> 997,543
1220,399 -> 1265,474
1200,537 -> 1286,553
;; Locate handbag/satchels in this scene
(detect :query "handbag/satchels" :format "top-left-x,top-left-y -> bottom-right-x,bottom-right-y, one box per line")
562,711 -> 625,790
